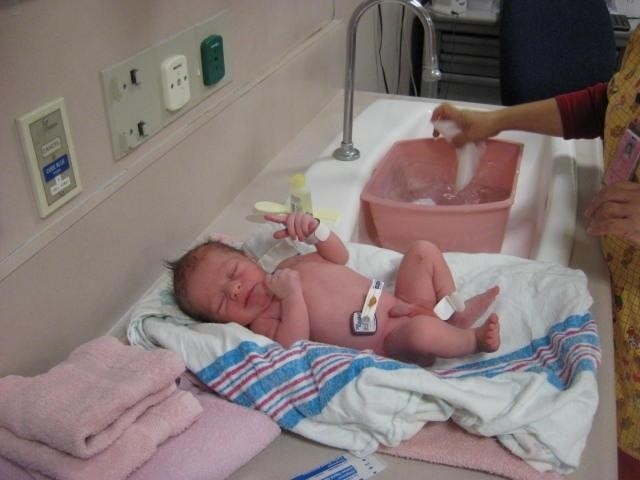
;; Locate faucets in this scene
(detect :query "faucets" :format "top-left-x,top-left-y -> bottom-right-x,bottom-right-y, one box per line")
332,0 -> 442,162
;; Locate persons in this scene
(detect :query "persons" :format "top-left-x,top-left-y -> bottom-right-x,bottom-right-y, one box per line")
163,211 -> 501,365
430,22 -> 640,480
496,0 -> 614,106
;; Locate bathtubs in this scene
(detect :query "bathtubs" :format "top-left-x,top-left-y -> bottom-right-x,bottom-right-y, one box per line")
282,96 -> 580,275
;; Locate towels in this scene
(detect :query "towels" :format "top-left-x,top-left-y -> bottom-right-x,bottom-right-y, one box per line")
0,333 -> 282,478
135,215 -> 593,479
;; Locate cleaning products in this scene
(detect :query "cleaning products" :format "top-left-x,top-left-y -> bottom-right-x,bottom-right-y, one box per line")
288,173 -> 313,216
